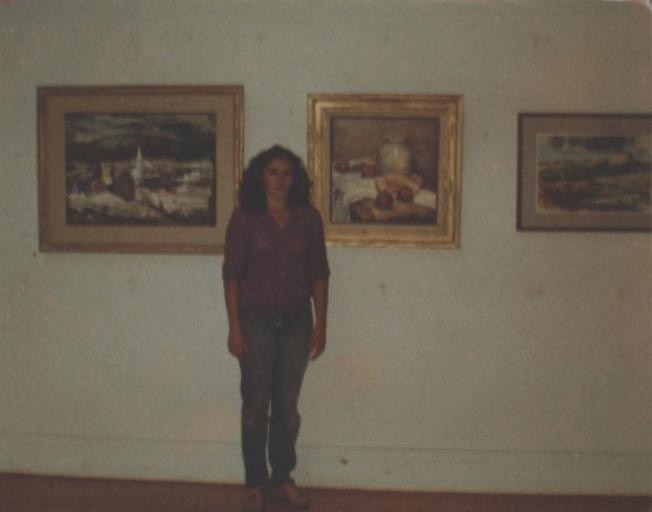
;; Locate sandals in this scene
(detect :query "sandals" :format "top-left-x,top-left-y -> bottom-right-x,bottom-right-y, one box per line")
270,477 -> 311,511
239,483 -> 267,511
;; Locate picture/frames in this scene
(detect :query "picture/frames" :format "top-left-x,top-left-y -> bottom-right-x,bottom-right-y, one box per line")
36,84 -> 247,256
305,90 -> 465,250
515,111 -> 652,233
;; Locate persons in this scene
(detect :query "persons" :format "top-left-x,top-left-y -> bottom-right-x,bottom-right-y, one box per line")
223,145 -> 330,511
